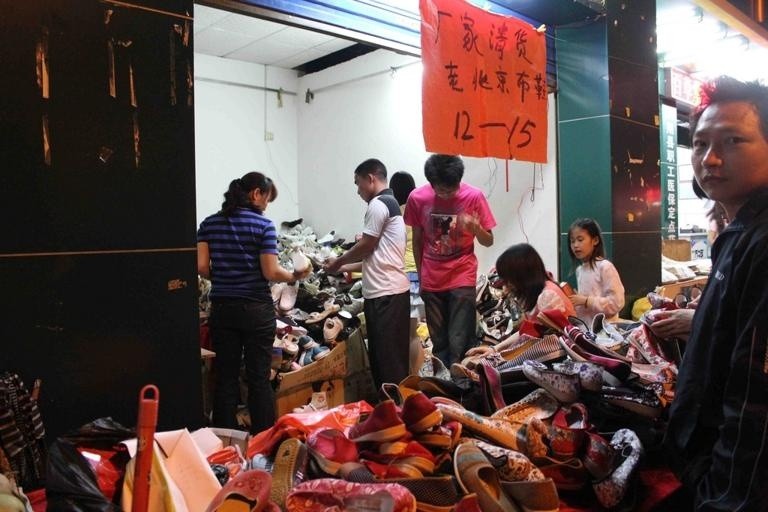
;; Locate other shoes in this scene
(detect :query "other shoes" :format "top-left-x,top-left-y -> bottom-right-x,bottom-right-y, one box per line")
200,232 -> 703,511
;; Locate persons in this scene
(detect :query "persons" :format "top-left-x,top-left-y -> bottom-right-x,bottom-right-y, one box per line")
389,170 -> 422,378
322,157 -> 414,403
649,176 -> 731,341
566,216 -> 626,328
658,73 -> 767,512
495,242 -> 576,326
196,173 -> 314,435
403,154 -> 496,376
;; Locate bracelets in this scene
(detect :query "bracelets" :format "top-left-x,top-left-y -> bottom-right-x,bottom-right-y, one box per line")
585,296 -> 588,308
